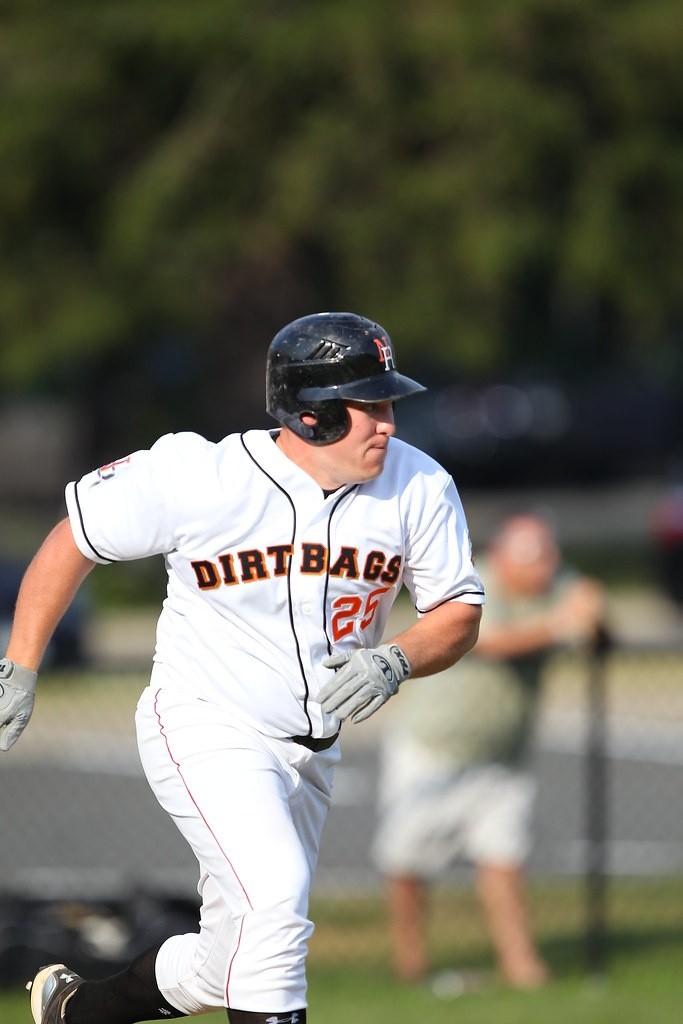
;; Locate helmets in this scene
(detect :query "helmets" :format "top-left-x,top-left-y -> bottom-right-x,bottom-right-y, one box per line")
264,312 -> 427,446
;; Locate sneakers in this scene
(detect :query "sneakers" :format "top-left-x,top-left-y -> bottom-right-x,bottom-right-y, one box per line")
29,961 -> 83,1024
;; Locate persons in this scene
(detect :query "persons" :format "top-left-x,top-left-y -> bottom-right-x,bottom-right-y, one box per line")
366,494 -> 611,994
1,309 -> 491,1024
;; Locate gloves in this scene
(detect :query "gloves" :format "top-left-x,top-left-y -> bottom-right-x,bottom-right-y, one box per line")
317,643 -> 412,725
0,657 -> 40,751
